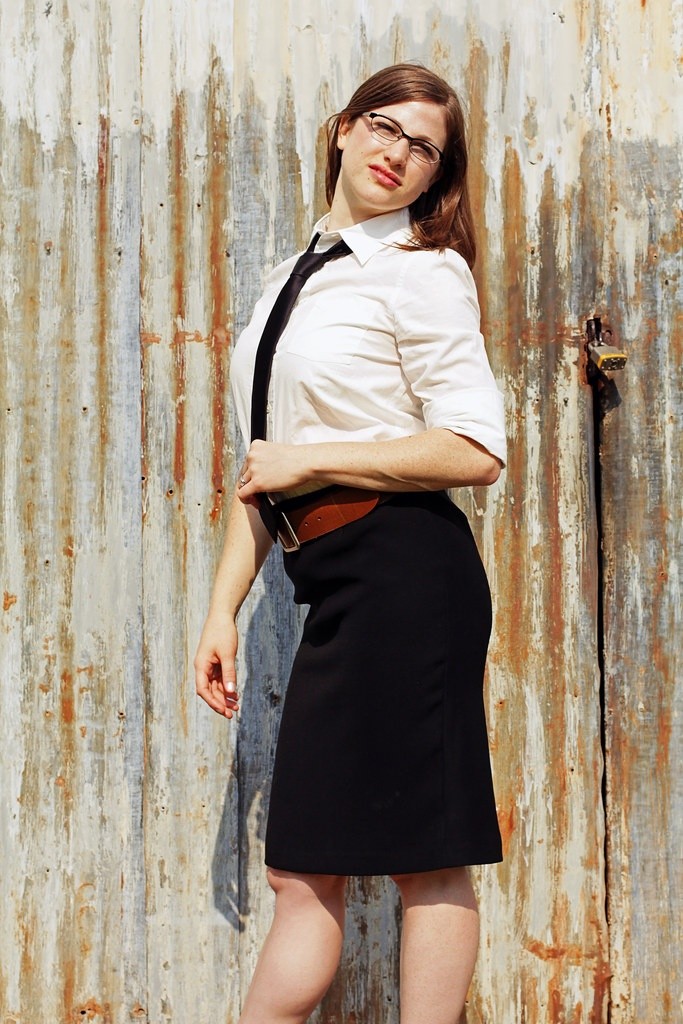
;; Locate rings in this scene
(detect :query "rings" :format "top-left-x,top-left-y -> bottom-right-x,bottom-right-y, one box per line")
240,477 -> 245,485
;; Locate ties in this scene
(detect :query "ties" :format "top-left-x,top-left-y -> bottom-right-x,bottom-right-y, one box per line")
251,232 -> 353,543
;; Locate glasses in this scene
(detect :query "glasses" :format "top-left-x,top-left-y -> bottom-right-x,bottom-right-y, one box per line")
361,111 -> 443,165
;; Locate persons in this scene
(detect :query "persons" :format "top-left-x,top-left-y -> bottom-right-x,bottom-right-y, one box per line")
194,63 -> 506,1024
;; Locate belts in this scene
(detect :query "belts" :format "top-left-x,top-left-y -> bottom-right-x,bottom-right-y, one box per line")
261,482 -> 399,553
